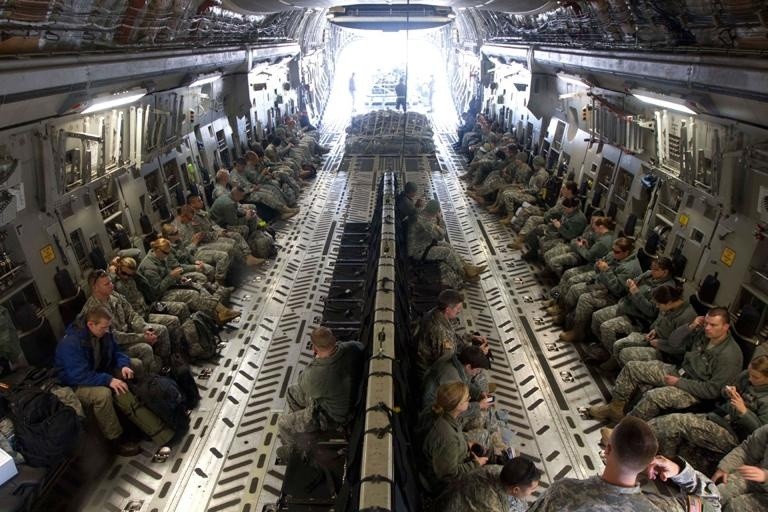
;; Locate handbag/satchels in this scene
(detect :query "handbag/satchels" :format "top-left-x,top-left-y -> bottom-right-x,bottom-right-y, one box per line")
173,354 -> 201,409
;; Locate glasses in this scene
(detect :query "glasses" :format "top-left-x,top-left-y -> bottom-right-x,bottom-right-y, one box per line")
93,271 -> 106,286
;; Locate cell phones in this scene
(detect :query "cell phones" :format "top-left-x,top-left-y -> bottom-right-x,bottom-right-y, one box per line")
485,397 -> 494,402
725,385 -> 732,391
267,167 -> 270,170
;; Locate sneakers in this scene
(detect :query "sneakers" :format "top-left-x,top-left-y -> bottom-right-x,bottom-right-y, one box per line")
102,438 -> 141,457
588,402 -> 625,422
458,170 -> 528,246
246,256 -> 265,266
535,271 -> 617,380
216,305 -> 242,321
216,285 -> 235,295
459,261 -> 488,282
280,143 -> 331,220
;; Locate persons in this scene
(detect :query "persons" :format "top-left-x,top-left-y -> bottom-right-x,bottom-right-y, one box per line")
399,112 -> 768,511
287,327 -> 366,425
1,112 -> 331,510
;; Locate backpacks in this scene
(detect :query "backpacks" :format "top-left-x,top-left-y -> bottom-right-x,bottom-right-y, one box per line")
182,311 -> 220,363
5,385 -> 82,469
249,231 -> 277,258
137,373 -> 190,432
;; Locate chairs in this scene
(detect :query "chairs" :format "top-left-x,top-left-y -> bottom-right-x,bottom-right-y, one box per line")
489,163 -> 767,384
272,188 -> 483,512
0,139 -> 272,512
